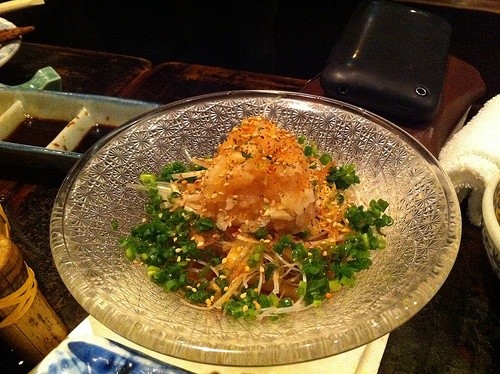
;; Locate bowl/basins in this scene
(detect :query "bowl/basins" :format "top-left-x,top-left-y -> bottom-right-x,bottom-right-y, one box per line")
481,174 -> 500,275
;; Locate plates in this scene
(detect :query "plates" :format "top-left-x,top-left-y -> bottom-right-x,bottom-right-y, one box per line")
0,18 -> 21,70
0,87 -> 159,161
49,89 -> 462,367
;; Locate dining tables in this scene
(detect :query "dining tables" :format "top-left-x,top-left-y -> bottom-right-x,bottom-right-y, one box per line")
0,42 -> 500,374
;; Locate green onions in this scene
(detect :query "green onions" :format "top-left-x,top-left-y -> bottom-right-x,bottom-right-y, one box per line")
111,115 -> 395,318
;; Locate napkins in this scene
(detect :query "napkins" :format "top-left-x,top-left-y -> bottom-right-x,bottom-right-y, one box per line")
439,93 -> 500,226
